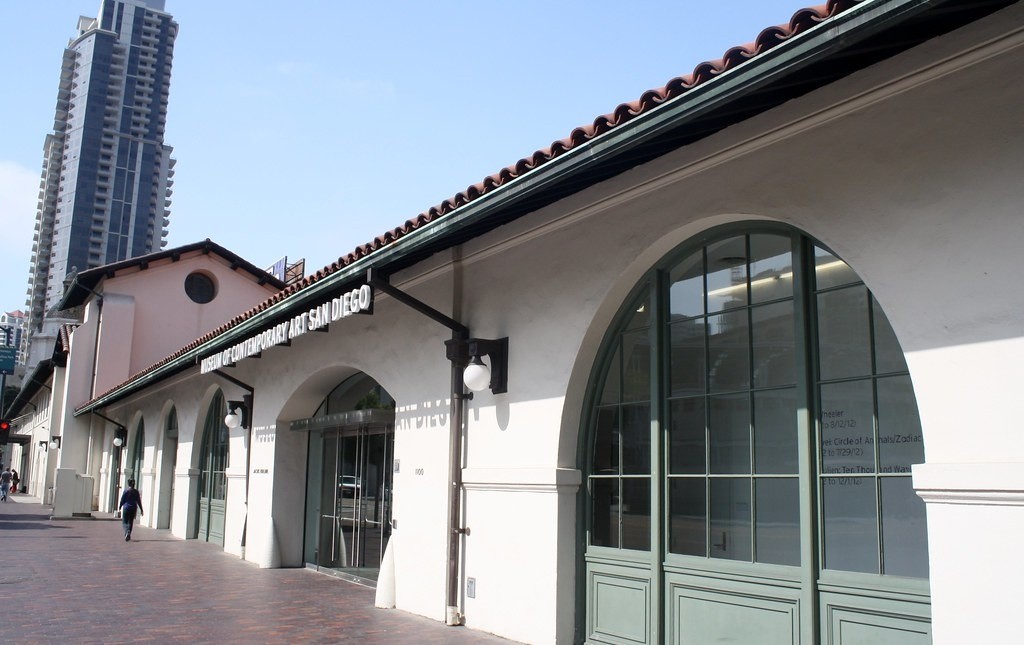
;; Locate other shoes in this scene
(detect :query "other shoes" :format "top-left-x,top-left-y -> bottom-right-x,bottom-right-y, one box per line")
125,531 -> 131,541
1,496 -> 4,501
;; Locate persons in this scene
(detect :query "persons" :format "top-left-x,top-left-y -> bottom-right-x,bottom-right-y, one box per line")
0,467 -> 12,501
118,478 -> 143,541
10,469 -> 20,493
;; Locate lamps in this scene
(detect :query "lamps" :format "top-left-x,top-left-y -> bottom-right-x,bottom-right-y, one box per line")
37,440 -> 48,451
113,428 -> 126,446
463,335 -> 509,393
225,400 -> 248,429
49,435 -> 61,449
